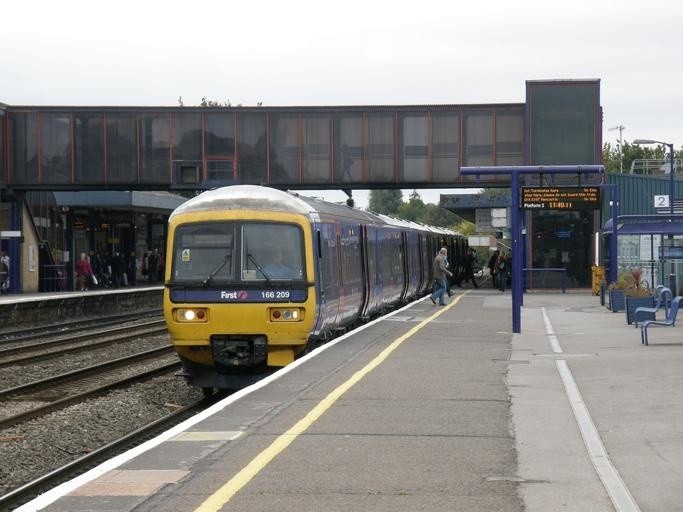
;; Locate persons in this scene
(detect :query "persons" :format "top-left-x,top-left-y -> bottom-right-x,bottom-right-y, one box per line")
489,251 -> 512,292
76,247 -> 161,291
439,251 -> 455,297
458,248 -> 481,288
1,250 -> 10,289
429,248 -> 453,306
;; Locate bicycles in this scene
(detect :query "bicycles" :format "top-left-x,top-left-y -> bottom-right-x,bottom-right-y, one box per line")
473,266 -> 483,279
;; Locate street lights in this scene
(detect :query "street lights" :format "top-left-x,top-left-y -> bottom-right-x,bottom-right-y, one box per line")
627,137 -> 675,298
616,124 -> 625,173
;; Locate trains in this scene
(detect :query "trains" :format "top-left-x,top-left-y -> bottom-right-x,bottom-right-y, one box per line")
163,183 -> 475,395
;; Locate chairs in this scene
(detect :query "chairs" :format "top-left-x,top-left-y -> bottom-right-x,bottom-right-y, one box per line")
606,272 -> 682,346
